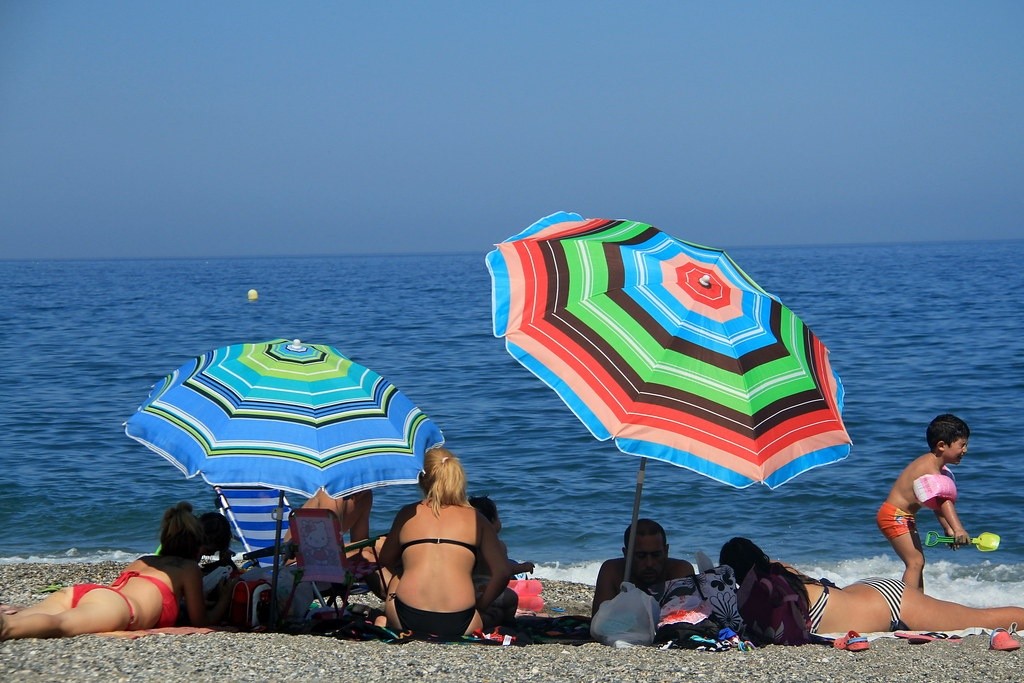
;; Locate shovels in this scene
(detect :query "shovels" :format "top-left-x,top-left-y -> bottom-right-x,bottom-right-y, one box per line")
924,530 -> 1001,553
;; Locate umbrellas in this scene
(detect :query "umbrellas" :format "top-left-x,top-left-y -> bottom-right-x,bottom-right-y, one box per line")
122,339 -> 445,626
485,210 -> 854,582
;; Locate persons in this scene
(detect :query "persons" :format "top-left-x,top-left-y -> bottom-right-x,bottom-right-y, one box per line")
0,502 -> 238,642
719,537 -> 1024,635
468,496 -> 534,628
374,447 -> 510,638
282,490 -> 394,602
877,414 -> 971,593
592,518 -> 695,621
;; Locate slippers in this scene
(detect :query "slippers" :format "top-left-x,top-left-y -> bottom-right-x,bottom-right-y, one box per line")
894,632 -> 963,644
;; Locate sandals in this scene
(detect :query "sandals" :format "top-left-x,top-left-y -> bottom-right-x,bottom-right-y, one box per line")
834,630 -> 869,650
989,627 -> 1020,650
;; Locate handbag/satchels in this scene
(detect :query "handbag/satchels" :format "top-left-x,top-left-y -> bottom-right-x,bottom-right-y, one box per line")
590,581 -> 661,647
737,563 -> 812,646
178,565 -> 314,629
647,564 -> 744,634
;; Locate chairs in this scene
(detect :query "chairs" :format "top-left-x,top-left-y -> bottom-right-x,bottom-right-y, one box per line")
213,485 -> 391,610
280,508 -> 359,635
